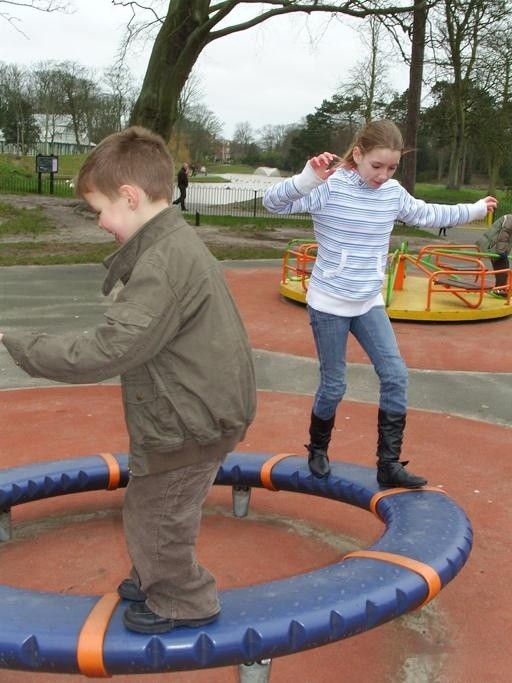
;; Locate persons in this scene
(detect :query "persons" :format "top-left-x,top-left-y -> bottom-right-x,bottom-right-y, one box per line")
0,122 -> 258,639
260,118 -> 498,491
172,162 -> 190,211
438,227 -> 446,236
471,212 -> 511,297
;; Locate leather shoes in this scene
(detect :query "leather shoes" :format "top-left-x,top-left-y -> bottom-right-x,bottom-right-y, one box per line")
120,576 -> 153,600
125,598 -> 218,633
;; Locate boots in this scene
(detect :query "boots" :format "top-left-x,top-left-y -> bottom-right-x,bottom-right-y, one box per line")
376,410 -> 428,489
305,408 -> 335,477
